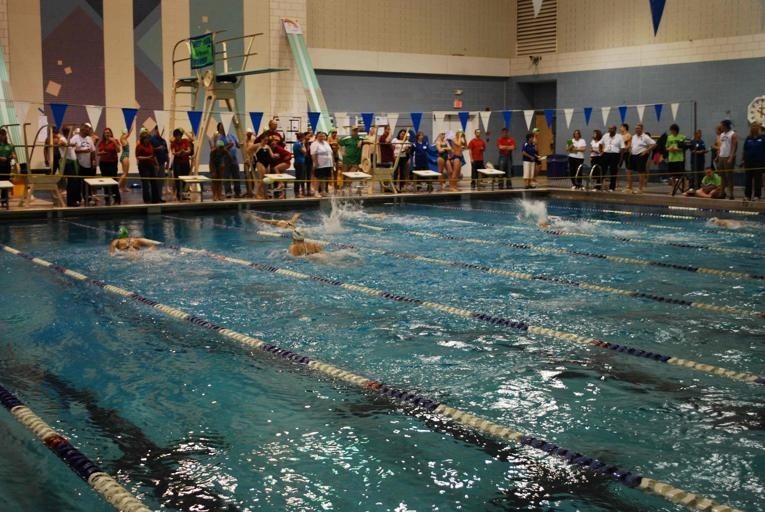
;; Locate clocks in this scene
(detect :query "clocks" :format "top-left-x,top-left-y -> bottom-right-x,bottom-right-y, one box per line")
744,94 -> 765,129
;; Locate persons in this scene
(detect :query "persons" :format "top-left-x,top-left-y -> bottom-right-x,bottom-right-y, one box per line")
741,122 -> 765,202
522,128 -> 542,189
467,128 -> 487,190
565,130 -> 587,189
293,125 -> 433,198
111,227 -> 156,263
665,121 -> 737,200
288,224 -> 331,264
2,128 -> 17,208
582,123 -> 657,194
243,211 -> 304,232
450,130 -> 467,191
135,125 -> 194,203
43,122 -> 134,207
209,120 -> 292,201
497,127 -> 516,190
536,215 -> 564,233
706,215 -> 747,230
434,130 -> 453,192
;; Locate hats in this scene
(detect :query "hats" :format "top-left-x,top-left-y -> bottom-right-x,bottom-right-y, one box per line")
330,127 -> 337,134
139,127 -> 149,138
216,140 -> 224,147
352,125 -> 358,130
